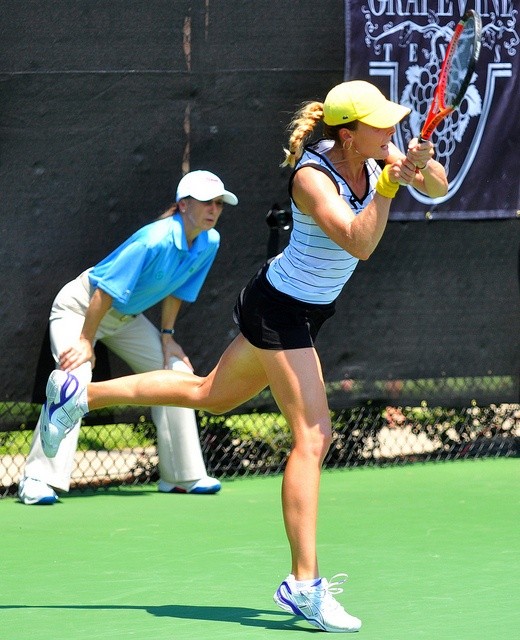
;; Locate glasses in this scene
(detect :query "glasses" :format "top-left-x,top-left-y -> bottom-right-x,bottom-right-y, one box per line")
200,198 -> 225,206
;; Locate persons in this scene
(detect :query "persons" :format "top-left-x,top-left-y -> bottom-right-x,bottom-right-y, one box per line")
38,79 -> 449,633
18,170 -> 239,506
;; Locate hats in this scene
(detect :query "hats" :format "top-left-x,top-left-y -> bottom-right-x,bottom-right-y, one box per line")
322,80 -> 413,129
176,170 -> 238,206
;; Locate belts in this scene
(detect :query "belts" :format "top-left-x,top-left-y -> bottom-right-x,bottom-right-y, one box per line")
81,267 -> 139,323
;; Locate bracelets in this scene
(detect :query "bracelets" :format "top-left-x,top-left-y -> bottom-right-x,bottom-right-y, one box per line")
374,162 -> 400,201
418,164 -> 429,171
162,329 -> 175,334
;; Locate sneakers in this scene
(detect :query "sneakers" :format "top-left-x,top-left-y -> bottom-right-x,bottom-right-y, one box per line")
157,476 -> 222,494
40,369 -> 85,457
272,573 -> 361,634
17,477 -> 58,505
